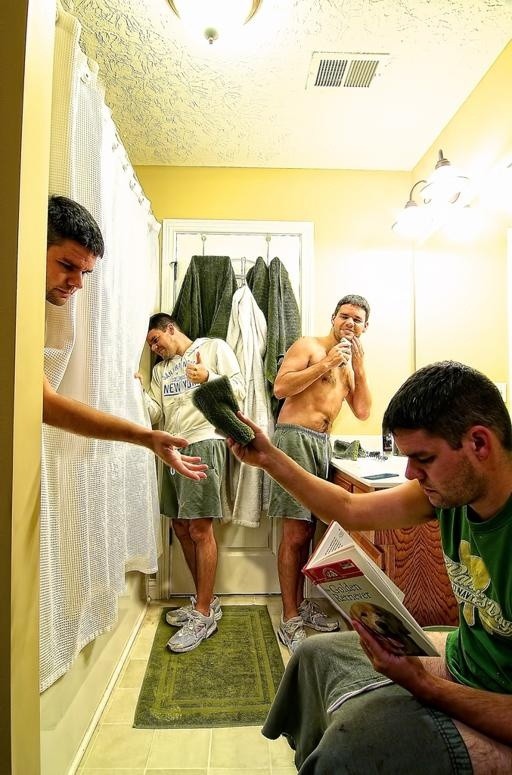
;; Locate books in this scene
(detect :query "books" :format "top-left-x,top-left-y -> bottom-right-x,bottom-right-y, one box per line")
302,518 -> 445,659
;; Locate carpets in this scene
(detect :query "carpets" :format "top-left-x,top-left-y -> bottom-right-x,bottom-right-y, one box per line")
132,604 -> 286,729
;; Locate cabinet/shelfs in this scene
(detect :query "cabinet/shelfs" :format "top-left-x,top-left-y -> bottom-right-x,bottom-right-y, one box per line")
322,471 -> 459,629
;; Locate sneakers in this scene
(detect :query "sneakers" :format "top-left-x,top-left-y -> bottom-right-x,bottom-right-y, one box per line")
278,612 -> 307,656
165,595 -> 223,627
166,609 -> 217,653
297,599 -> 338,632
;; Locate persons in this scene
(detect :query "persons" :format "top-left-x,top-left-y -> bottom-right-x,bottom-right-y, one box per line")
227,360 -> 512,774
133,313 -> 245,656
269,293 -> 375,655
46,196 -> 210,485
